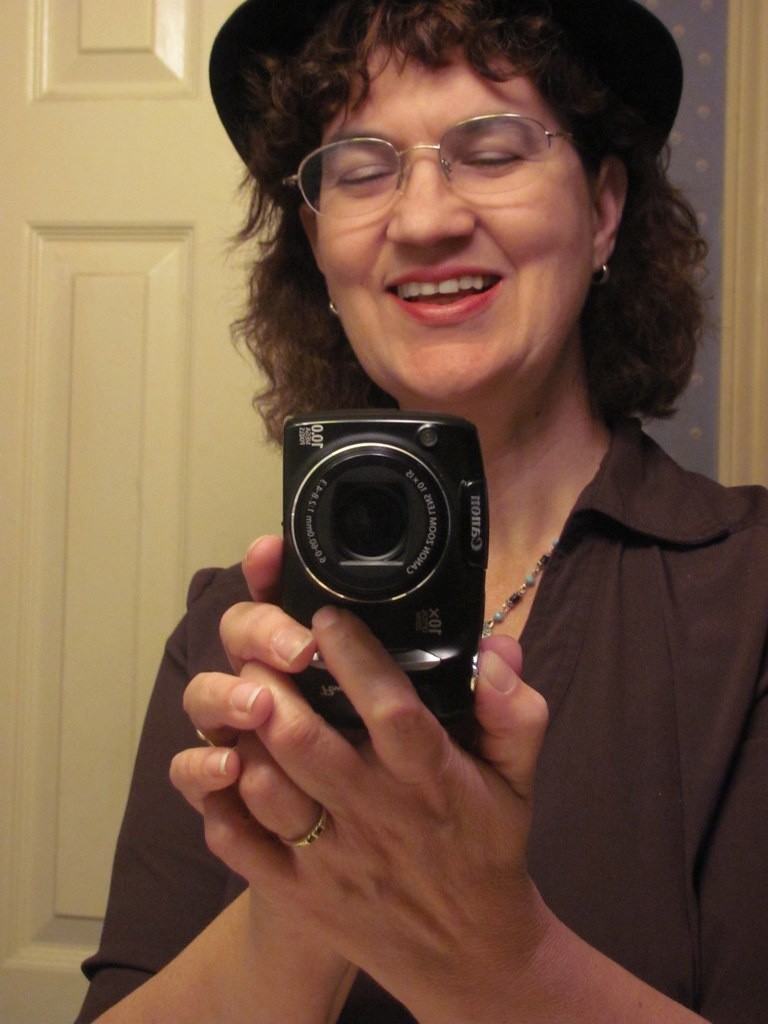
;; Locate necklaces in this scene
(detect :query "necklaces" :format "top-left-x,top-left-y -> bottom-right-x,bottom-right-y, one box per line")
482,536 -> 559,638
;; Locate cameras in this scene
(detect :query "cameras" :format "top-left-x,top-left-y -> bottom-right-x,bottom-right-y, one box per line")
281,408 -> 491,751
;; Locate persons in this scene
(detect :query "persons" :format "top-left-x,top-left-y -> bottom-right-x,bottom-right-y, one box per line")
74,0 -> 768,1024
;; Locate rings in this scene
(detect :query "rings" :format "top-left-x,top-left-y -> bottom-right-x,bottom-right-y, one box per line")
279,807 -> 328,846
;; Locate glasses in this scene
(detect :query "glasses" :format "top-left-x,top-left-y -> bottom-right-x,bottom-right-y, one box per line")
281,113 -> 577,218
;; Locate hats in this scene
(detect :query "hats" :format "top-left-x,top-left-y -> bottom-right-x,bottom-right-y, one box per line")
209,0 -> 684,206
